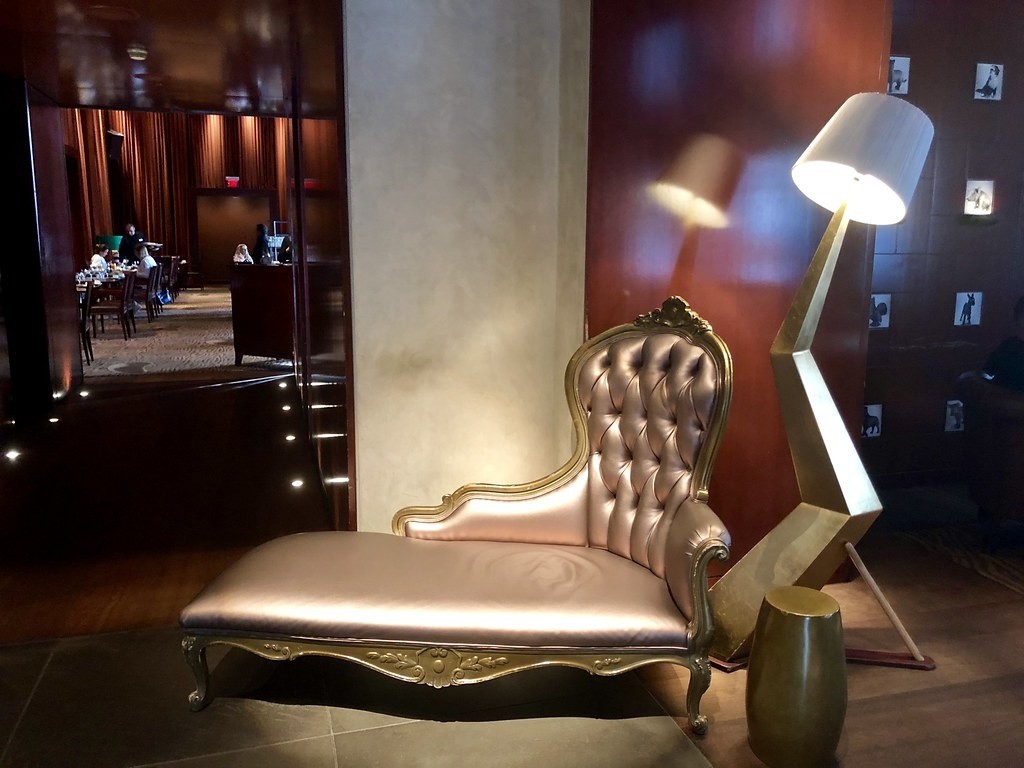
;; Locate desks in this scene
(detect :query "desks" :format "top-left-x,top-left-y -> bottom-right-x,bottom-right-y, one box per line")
76,270 -> 125,282
76,281 -> 104,323
107,265 -> 142,272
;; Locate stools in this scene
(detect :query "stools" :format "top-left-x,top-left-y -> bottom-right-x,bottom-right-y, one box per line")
747,586 -> 849,768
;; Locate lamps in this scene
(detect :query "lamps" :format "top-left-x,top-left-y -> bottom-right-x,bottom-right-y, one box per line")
692,89 -> 935,674
127,40 -> 148,60
645,134 -> 747,301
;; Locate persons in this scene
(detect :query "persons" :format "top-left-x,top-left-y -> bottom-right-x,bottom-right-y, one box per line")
90,224 -> 157,315
250,224 -> 292,263
233,244 -> 254,264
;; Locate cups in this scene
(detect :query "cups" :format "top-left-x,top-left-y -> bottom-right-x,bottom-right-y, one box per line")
76,256 -> 127,280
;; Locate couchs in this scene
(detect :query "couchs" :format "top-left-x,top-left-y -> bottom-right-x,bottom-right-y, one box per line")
945,362 -> 1024,552
181,293 -> 736,738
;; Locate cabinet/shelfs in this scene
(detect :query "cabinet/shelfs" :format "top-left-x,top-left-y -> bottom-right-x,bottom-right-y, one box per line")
231,263 -> 295,366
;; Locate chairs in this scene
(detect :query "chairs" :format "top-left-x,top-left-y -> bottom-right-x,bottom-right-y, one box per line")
77,255 -> 208,367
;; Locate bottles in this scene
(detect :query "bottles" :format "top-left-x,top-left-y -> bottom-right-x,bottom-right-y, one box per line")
260,249 -> 270,265
